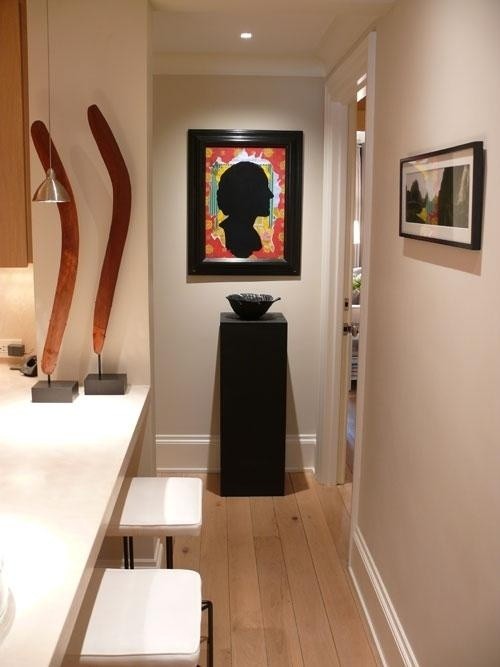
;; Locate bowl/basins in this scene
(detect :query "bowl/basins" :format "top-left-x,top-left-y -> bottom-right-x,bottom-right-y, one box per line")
226,293 -> 281,321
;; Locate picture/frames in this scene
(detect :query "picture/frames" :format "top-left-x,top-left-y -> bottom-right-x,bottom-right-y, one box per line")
399,140 -> 484,250
187,128 -> 303,277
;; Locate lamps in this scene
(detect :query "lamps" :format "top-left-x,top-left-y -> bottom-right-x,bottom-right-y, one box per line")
31,0 -> 70,203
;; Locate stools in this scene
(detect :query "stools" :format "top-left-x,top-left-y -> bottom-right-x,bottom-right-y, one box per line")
105,477 -> 204,569
61,568 -> 213,667
